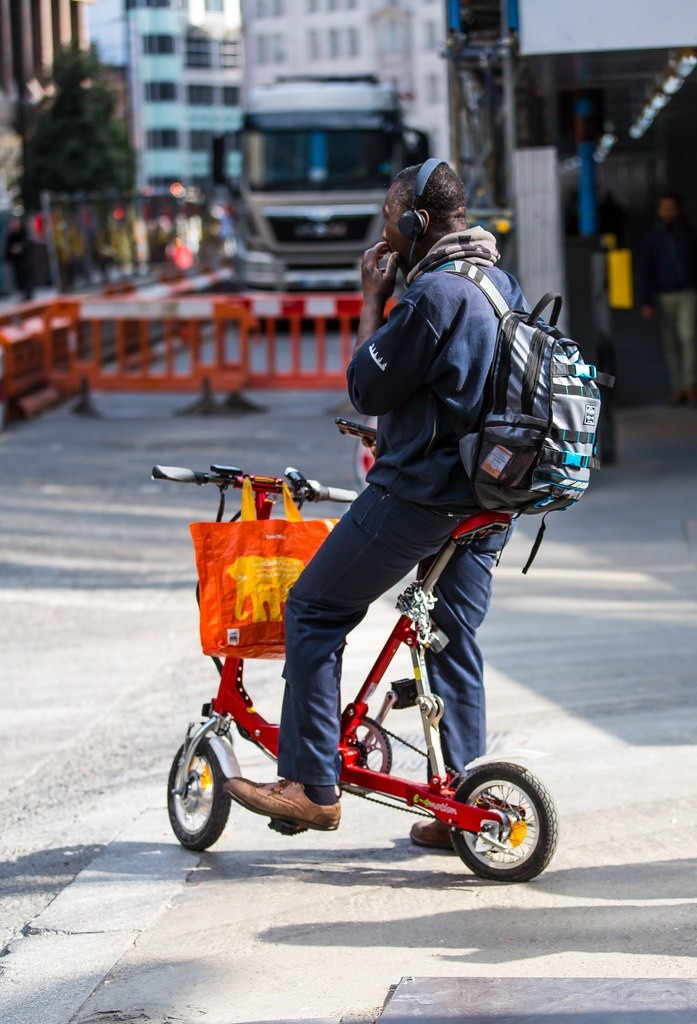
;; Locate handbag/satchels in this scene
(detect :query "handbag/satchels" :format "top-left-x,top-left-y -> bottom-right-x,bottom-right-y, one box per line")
191,477 -> 341,661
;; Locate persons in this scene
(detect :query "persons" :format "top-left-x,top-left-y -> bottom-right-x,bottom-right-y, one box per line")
221,162 -> 529,853
642,197 -> 697,409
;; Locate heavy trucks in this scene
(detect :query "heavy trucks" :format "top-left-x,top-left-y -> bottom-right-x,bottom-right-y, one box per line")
212,78 -> 431,330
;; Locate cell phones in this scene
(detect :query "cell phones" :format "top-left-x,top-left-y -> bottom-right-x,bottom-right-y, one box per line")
335,417 -> 377,442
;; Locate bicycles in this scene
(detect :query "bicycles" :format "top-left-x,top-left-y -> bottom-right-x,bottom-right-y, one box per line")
149,465 -> 560,884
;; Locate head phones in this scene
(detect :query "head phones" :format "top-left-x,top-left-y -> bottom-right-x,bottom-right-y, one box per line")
398,158 -> 450,242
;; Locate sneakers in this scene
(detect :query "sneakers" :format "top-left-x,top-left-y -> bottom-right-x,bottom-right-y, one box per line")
223,777 -> 341,831
411,819 -> 456,852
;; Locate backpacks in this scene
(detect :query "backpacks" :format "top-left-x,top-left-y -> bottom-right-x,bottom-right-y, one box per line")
432,259 -> 615,518
